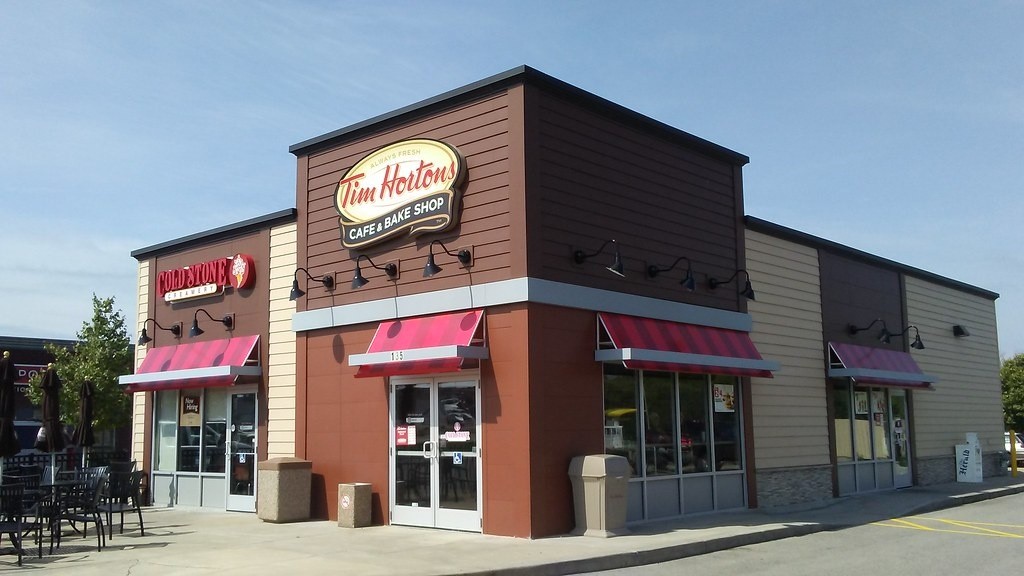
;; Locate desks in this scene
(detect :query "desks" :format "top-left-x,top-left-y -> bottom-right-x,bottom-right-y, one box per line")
38,480 -> 88,548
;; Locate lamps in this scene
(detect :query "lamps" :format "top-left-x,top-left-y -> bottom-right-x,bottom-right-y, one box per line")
850,319 -> 891,343
708,269 -> 755,300
351,254 -> 397,289
650,257 -> 696,293
576,238 -> 626,277
189,309 -> 232,338
889,325 -> 925,349
289,267 -> 333,300
138,318 -> 180,346
423,239 -> 471,277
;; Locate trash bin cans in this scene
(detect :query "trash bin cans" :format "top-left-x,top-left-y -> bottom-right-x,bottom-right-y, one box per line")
955,445 -> 983,483
337,482 -> 372,528
568,454 -> 632,538
257,456 -> 313,524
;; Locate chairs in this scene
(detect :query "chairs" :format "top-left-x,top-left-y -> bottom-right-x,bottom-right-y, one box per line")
395,446 -> 472,501
0,460 -> 143,566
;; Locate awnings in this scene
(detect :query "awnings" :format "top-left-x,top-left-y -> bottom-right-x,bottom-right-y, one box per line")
599,312 -> 774,379
829,341 -> 932,388
354,308 -> 486,378
126,334 -> 261,393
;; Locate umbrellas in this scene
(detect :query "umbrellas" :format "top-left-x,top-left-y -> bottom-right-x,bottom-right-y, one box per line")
0,350 -> 24,485
70,380 -> 96,487
33,362 -> 70,504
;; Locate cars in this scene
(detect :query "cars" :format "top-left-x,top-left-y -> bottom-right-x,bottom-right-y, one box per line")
1005,432 -> 1024,467
184,424 -> 253,466
645,417 -> 704,472
